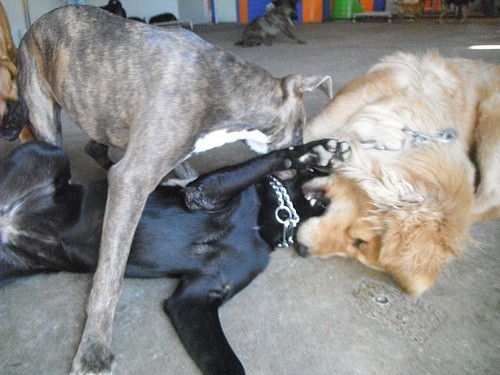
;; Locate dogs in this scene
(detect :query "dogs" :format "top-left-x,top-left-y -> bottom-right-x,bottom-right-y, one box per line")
18,5 -> 333,375
0,138 -> 351,375
296,50 -> 500,295
233,0 -> 306,48
0,2 -> 35,143
